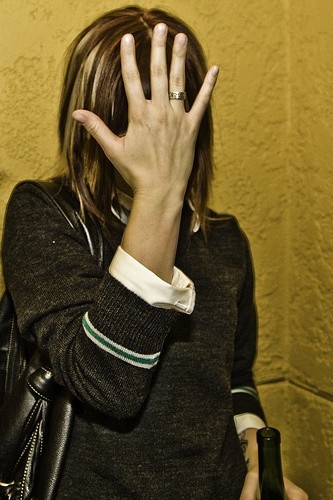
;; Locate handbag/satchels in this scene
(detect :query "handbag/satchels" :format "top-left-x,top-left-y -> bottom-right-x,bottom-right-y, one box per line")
0,180 -> 104,500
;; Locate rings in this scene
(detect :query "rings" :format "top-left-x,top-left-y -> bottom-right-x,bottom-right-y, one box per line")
168,92 -> 186,100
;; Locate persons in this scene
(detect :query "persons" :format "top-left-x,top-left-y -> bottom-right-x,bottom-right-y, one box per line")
0,5 -> 308,500
